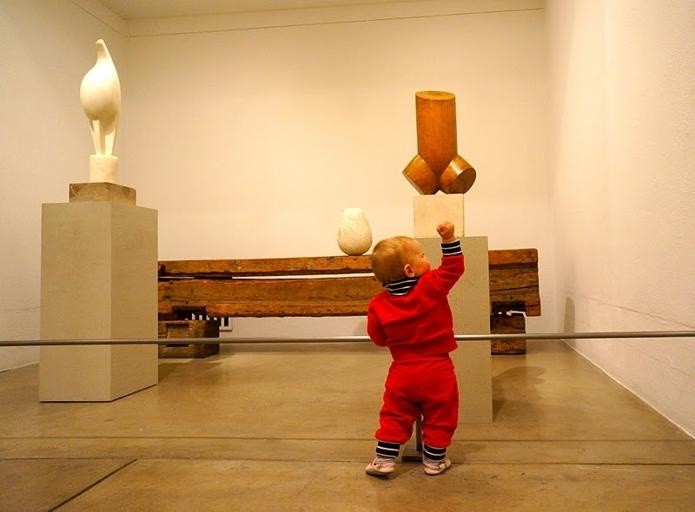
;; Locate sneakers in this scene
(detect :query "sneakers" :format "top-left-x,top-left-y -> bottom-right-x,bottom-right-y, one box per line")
365,457 -> 394,476
423,456 -> 451,474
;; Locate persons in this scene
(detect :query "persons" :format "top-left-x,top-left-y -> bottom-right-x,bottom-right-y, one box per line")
365,221 -> 466,475
